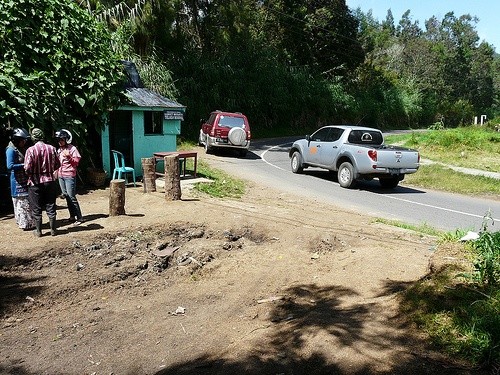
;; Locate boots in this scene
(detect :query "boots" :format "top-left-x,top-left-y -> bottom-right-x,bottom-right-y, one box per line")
35,218 -> 43,236
49,216 -> 58,236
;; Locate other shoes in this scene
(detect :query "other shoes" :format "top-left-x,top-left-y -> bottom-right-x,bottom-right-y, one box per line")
62,218 -> 74,223
74,221 -> 81,225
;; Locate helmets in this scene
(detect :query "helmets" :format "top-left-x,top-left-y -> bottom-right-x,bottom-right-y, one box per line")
55,129 -> 72,144
13,128 -> 29,139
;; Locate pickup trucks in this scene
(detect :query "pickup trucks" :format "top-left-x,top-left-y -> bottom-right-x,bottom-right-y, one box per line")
288,125 -> 421,189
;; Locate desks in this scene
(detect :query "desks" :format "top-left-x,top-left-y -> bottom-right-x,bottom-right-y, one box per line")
153,151 -> 198,179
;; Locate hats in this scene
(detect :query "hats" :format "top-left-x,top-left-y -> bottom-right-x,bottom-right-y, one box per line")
31,128 -> 44,141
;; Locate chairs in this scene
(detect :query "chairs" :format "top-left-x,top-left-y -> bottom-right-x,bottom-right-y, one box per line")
111,149 -> 136,188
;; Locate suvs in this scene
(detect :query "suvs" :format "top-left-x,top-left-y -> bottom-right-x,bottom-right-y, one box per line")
198,109 -> 251,155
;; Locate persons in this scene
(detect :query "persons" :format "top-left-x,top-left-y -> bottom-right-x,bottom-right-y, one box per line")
55,129 -> 84,226
6,125 -> 36,230
23,128 -> 59,237
